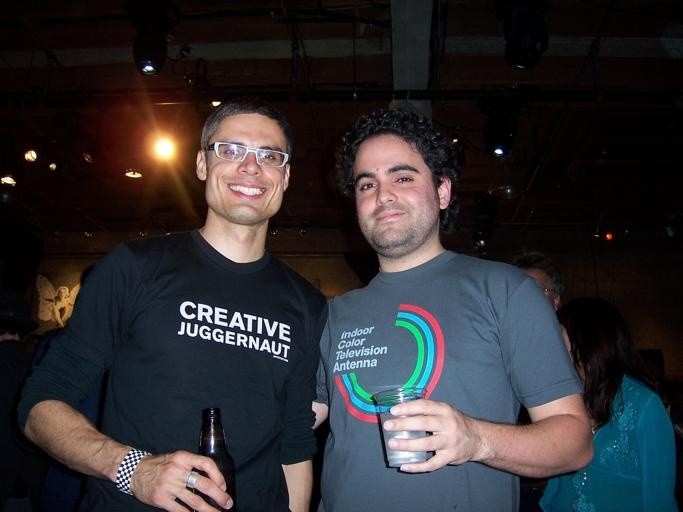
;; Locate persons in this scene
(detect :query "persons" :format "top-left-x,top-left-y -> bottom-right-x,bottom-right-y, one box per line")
16,96 -> 329,512
0,289 -> 52,512
509,254 -> 563,512
311,108 -> 594,512
537,297 -> 679,512
45,286 -> 74,328
31,264 -> 105,512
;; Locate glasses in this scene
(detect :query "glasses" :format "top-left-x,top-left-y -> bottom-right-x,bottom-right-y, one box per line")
206,141 -> 290,168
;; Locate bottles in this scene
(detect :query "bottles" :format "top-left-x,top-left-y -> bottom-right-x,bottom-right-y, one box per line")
192,407 -> 237,512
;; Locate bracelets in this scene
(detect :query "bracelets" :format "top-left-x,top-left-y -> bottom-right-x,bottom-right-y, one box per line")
113,448 -> 152,496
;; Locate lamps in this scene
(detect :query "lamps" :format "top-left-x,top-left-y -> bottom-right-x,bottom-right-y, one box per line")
477,95 -> 522,159
506,11 -> 549,72
472,190 -> 498,257
127,0 -> 181,76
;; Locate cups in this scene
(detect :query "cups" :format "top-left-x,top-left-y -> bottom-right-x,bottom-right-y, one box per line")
371,387 -> 427,468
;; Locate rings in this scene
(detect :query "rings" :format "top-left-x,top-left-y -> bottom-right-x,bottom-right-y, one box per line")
187,471 -> 198,488
